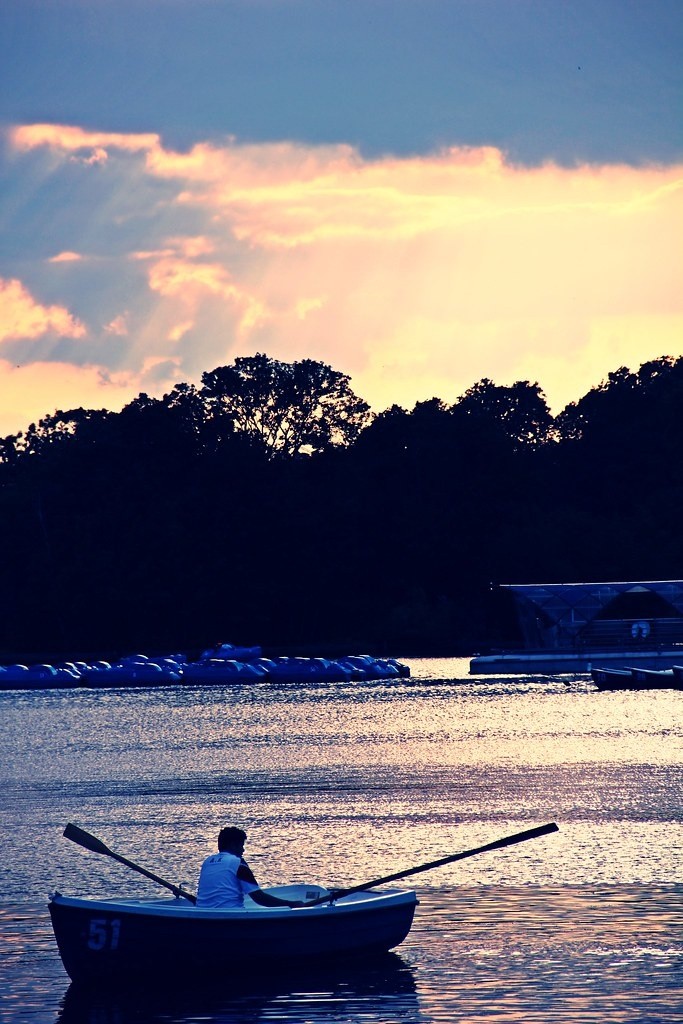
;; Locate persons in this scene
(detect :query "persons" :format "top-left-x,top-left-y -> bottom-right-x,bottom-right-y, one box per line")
194,827 -> 304,908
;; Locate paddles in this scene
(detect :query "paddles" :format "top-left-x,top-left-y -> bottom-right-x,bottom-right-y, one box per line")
304,822 -> 559,908
62,821 -> 197,903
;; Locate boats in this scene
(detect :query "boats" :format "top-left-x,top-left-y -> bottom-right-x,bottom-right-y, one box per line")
45,885 -> 421,986
669,665 -> 683,690
589,664 -> 632,690
0,642 -> 411,689
624,666 -> 675,690
